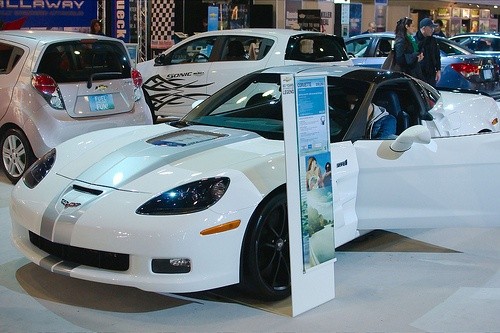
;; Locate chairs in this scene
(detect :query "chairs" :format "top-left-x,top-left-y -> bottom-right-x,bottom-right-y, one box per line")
226,41 -> 248,60
41,48 -> 65,75
379,40 -> 392,56
376,90 -> 410,136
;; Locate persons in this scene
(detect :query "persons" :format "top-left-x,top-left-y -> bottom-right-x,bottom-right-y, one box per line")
307,157 -> 332,190
433,19 -> 446,38
414,18 -> 442,89
393,17 -> 425,82
342,86 -> 397,140
87,21 -> 110,66
362,21 -> 377,34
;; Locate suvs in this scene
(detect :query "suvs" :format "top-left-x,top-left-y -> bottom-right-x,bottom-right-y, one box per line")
133,27 -> 348,122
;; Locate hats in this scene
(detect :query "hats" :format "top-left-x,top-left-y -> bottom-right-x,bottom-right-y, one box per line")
420,18 -> 438,28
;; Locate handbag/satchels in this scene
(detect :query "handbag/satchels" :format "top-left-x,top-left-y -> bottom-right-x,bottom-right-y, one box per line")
380,50 -> 401,72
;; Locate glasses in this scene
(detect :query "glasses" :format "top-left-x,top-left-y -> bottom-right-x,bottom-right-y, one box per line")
405,17 -> 408,23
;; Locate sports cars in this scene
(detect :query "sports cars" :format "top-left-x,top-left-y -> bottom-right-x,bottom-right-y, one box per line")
7,63 -> 500,304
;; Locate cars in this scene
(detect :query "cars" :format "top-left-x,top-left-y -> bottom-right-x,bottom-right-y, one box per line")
451,34 -> 500,52
341,30 -> 500,99
0,31 -> 152,186
